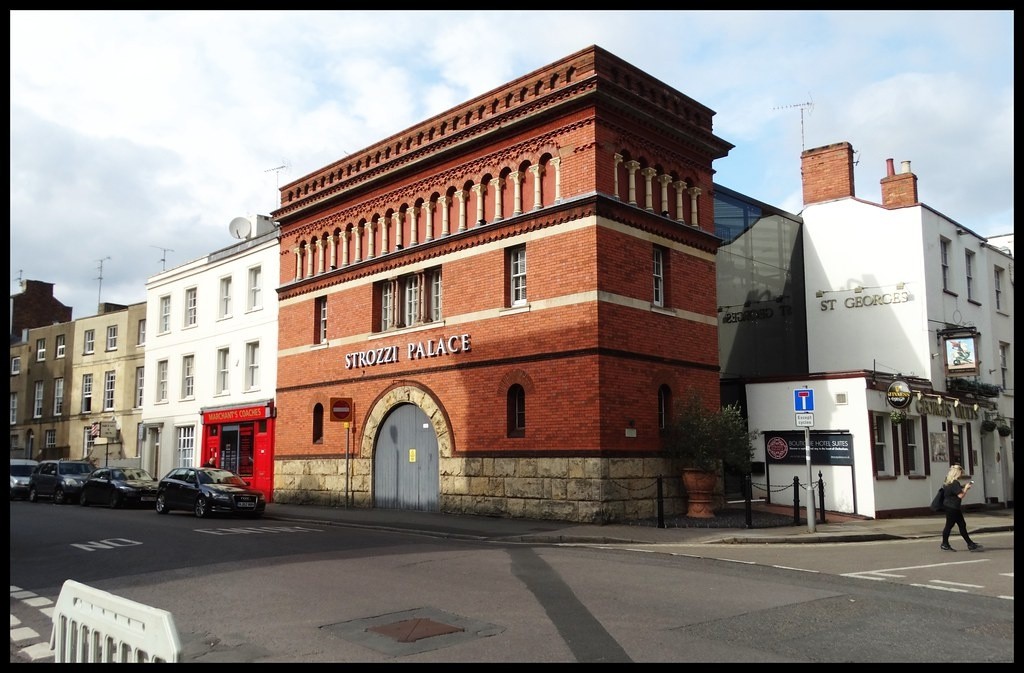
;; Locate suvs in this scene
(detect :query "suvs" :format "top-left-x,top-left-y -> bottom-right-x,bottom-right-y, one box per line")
28,458 -> 102,505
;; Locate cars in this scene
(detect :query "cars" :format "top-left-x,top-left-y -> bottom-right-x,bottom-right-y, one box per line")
154,466 -> 266,520
78,467 -> 161,510
10,458 -> 39,498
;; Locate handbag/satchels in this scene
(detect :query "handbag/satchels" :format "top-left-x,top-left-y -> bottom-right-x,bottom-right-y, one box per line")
930,488 -> 945,514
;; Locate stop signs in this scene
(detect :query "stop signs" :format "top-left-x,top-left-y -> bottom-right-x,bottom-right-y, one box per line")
331,400 -> 352,420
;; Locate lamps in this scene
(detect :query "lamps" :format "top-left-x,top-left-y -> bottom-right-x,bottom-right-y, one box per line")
913,390 -> 924,400
946,396 -> 961,408
896,282 -> 908,290
967,402 -> 979,412
925,393 -> 944,405
854,285 -> 869,293
957,229 -> 963,236
264,406 -> 277,419
979,242 -> 986,248
815,289 -> 829,299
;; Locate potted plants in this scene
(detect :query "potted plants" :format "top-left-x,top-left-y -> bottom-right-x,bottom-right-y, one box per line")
660,393 -> 758,520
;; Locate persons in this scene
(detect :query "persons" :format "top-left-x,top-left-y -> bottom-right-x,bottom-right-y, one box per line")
940,465 -> 983,552
203,458 -> 217,468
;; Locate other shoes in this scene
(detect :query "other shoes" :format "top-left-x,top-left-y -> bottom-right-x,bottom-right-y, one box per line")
968,544 -> 984,551
941,544 -> 956,552
